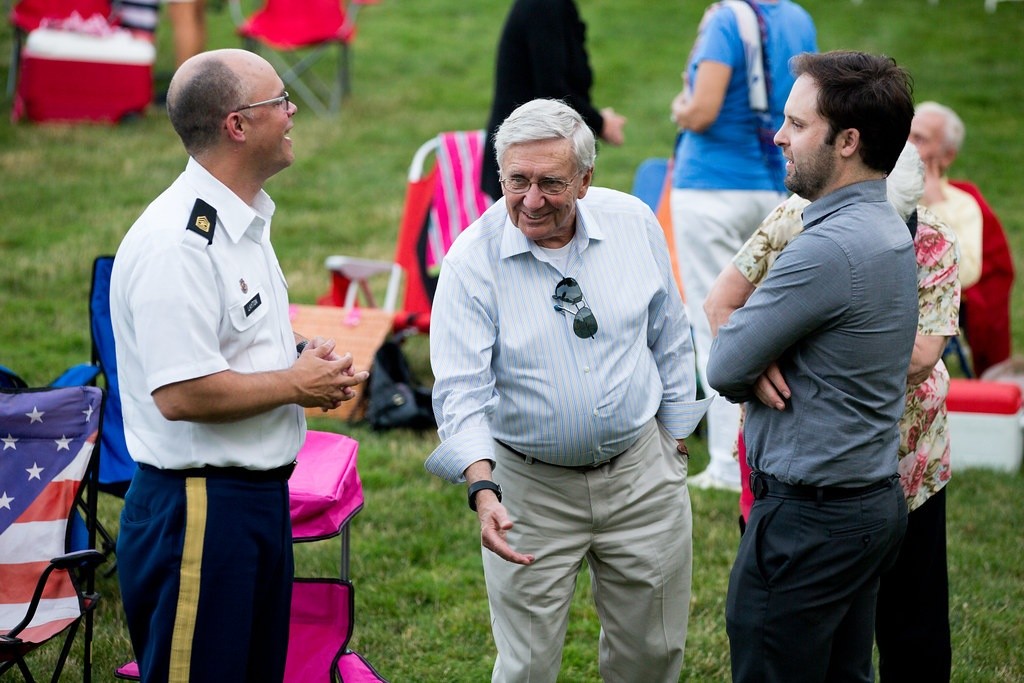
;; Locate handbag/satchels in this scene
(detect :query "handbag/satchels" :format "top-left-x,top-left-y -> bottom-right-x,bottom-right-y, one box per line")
365,348 -> 435,427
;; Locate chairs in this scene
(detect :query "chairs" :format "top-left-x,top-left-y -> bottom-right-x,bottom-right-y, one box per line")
228,0 -> 373,110
328,133 -> 496,349
0,388 -> 103,683
116,430 -> 385,683
0,255 -> 136,577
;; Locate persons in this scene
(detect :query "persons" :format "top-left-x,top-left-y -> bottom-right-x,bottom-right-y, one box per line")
896,101 -> 981,286
706,51 -> 925,683
426,97 -> 715,683
705,144 -> 961,683
671,0 -> 819,494
109,48 -> 367,683
475,0 -> 626,205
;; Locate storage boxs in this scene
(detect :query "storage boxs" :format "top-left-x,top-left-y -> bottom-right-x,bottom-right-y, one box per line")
17,28 -> 154,128
946,378 -> 1024,477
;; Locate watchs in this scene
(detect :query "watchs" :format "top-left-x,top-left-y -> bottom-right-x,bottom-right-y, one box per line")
464,481 -> 504,512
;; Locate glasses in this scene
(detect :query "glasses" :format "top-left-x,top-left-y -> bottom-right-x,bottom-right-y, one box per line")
552,275 -> 598,340
497,165 -> 587,195
224,91 -> 290,131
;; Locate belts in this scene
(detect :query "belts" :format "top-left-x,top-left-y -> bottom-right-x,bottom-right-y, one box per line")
165,461 -> 296,481
496,437 -> 626,472
749,471 -> 895,503
